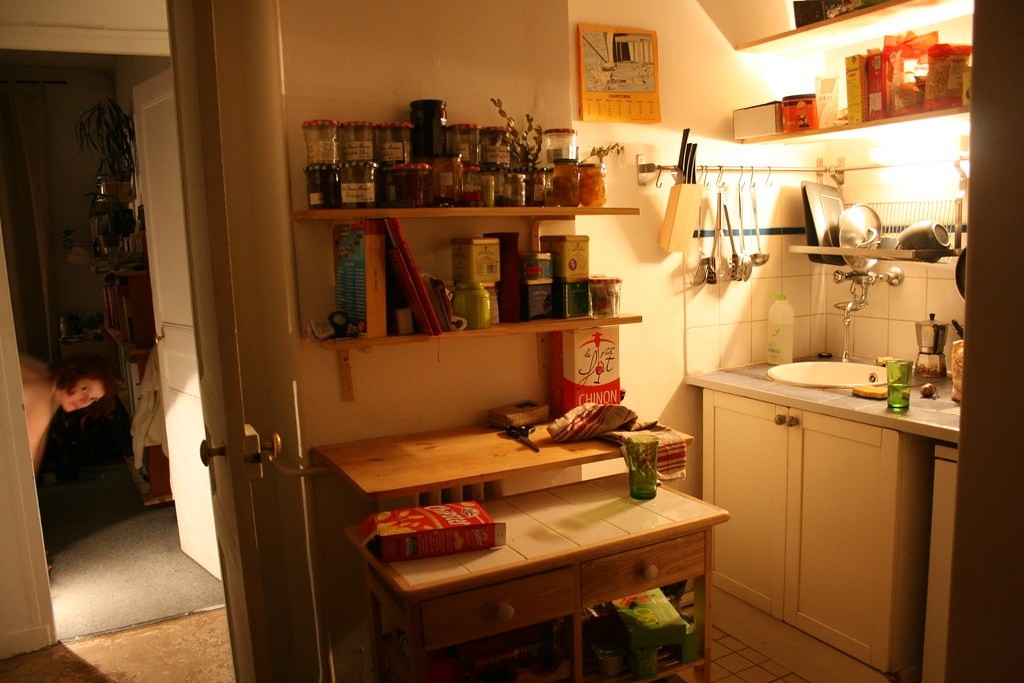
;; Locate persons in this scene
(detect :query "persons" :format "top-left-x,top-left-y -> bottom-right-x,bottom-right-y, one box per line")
19,352 -> 114,480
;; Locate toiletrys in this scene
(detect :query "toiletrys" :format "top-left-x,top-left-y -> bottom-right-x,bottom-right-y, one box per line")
767,293 -> 794,365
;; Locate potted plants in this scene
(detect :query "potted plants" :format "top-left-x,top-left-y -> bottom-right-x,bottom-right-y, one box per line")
72,93 -> 138,259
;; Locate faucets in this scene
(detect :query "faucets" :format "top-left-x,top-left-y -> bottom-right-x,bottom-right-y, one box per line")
832,266 -> 904,326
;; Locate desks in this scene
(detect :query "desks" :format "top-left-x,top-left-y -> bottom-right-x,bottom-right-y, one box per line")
310,421 -> 697,513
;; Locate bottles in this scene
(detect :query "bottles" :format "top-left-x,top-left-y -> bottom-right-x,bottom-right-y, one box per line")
767,293 -> 794,365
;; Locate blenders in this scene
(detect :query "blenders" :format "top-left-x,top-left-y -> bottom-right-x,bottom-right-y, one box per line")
913,313 -> 949,377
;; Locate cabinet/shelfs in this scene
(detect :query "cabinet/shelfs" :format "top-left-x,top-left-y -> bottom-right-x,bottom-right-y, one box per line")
733,0 -> 972,146
702,387 -> 936,674
101,263 -> 175,508
293,207 -> 644,402
351,472 -> 731,683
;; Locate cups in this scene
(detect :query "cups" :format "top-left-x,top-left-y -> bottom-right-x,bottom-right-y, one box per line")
885,358 -> 914,411
625,435 -> 659,499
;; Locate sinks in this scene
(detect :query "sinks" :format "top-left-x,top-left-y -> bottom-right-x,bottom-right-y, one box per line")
767,361 -> 888,388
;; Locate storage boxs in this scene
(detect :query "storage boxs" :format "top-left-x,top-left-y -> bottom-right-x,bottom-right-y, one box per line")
334,216 -> 387,340
612,629 -> 657,680
605,587 -> 686,649
847,32 -> 900,125
549,325 -> 621,420
442,623 -> 555,678
346,499 -> 507,565
732,100 -> 784,139
662,618 -> 700,663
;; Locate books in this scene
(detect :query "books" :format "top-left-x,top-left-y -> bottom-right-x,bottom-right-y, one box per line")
99,230 -> 149,420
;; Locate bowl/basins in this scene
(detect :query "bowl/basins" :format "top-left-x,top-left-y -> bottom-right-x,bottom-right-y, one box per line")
898,220 -> 950,263
